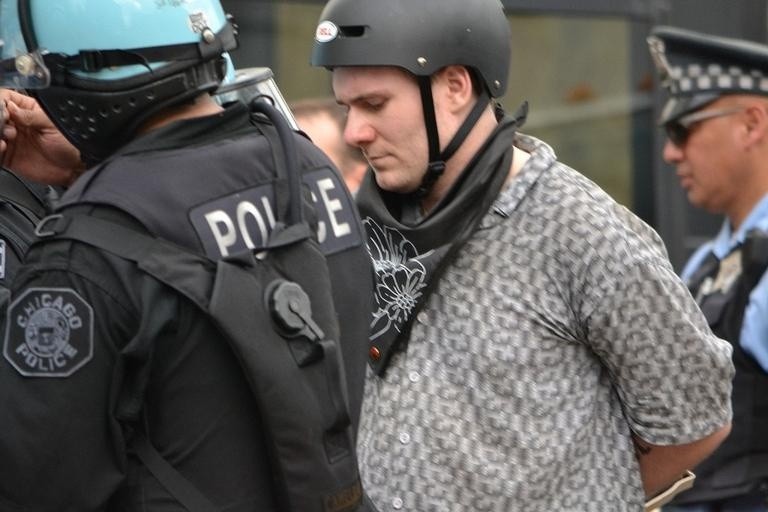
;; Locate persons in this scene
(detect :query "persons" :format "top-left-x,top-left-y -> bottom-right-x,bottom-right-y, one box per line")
646,26 -> 766,512
308,1 -> 738,511
1,1 -> 377,510
290,94 -> 369,198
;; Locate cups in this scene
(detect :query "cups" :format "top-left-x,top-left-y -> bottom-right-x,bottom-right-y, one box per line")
664,104 -> 742,145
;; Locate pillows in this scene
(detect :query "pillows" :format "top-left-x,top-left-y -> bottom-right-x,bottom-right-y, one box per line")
310,0 -> 512,98
0,0 -> 240,165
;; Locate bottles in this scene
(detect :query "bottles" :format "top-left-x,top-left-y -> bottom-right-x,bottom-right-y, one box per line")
643,22 -> 768,127
